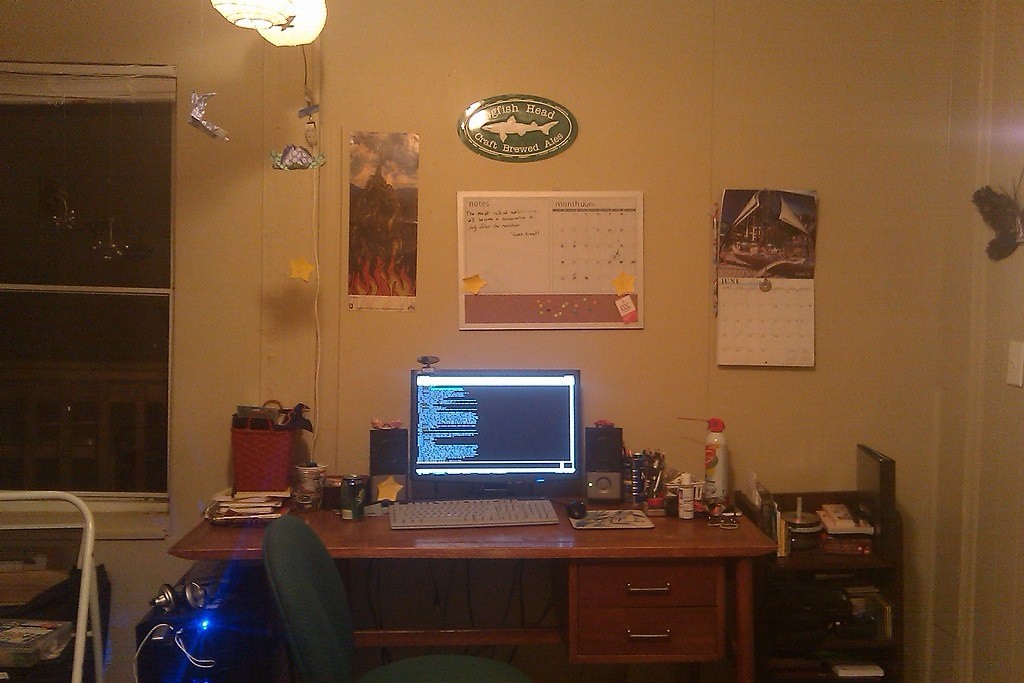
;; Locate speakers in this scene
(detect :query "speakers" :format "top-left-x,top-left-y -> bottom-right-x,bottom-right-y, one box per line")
369,429 -> 408,506
584,427 -> 623,502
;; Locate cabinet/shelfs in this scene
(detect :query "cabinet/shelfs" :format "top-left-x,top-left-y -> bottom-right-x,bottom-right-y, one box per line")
169,503 -> 779,683
736,489 -> 905,683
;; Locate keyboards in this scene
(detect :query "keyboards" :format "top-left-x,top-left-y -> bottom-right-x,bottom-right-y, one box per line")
388,498 -> 559,530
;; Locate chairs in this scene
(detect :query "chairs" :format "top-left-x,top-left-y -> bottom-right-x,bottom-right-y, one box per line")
261,514 -> 533,683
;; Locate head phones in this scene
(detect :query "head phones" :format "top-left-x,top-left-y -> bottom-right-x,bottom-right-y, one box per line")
149,576 -> 216,612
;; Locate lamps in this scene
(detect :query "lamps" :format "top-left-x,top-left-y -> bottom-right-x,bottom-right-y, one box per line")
209,0 -> 328,48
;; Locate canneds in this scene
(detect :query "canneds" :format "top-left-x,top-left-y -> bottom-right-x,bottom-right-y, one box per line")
341,473 -> 363,520
678,485 -> 694,519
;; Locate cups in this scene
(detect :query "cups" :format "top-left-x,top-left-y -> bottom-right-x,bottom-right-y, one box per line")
295,463 -> 326,511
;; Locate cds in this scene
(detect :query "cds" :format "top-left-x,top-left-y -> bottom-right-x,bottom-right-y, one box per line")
783,511 -> 824,549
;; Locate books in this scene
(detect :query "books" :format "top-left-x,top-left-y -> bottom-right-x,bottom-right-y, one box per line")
832,664 -> 884,677
816,505 -> 874,535
211,486 -> 291,527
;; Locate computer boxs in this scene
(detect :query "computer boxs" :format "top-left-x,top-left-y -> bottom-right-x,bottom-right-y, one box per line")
134,559 -> 294,683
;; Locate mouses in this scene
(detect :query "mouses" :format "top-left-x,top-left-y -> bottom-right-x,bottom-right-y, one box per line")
567,500 -> 586,519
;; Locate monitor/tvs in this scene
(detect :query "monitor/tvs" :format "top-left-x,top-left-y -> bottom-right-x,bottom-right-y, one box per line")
408,369 -> 585,499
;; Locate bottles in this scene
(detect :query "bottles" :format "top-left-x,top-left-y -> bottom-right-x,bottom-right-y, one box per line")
678,474 -> 695,520
